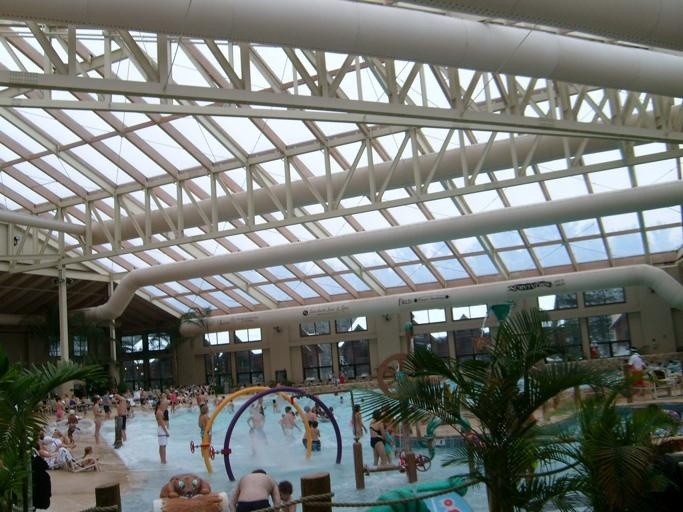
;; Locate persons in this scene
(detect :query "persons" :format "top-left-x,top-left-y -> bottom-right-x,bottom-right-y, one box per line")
625,345 -> 647,397
25,382 -> 397,511
645,403 -> 681,429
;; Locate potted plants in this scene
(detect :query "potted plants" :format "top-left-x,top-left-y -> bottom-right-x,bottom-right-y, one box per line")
347,305 -> 647,512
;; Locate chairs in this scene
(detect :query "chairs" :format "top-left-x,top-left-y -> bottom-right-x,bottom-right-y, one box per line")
41,385 -> 213,415
649,366 -> 673,398
59,450 -> 100,471
38,443 -> 64,470
